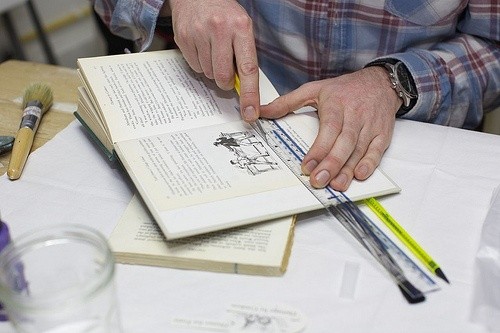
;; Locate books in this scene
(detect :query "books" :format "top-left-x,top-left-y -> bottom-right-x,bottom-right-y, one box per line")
105,190 -> 300,277
73,45 -> 402,242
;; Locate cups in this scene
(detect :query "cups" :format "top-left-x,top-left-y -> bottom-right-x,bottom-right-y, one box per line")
0,223 -> 124,333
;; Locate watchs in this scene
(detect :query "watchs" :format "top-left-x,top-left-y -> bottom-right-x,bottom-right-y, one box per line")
364,59 -> 418,117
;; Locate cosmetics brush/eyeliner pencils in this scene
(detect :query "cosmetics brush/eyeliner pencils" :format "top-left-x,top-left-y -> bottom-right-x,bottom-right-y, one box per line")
6,81 -> 53,181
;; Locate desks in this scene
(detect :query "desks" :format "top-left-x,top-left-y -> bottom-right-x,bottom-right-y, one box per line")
1,59 -> 500,333
